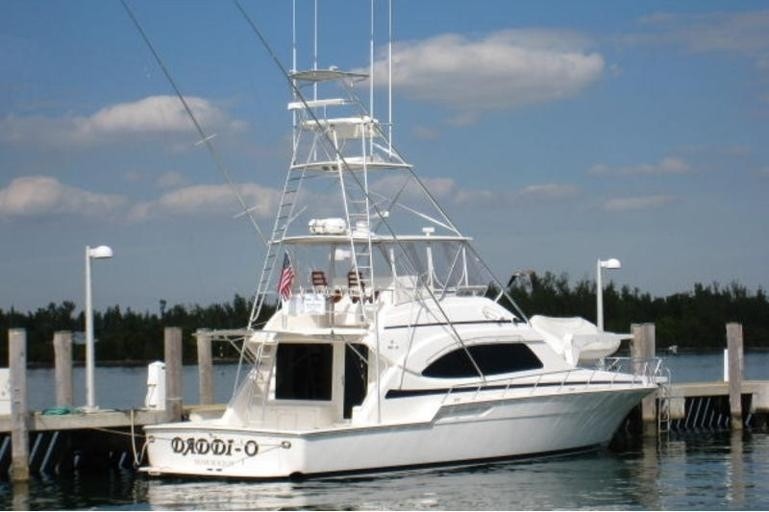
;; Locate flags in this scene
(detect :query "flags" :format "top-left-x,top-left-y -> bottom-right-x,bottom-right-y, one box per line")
277,250 -> 296,303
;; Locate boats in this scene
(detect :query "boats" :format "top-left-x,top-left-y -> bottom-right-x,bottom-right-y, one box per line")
121,0 -> 669,482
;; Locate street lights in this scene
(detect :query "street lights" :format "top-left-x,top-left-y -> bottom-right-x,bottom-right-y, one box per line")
596,258 -> 621,371
79,244 -> 113,412
